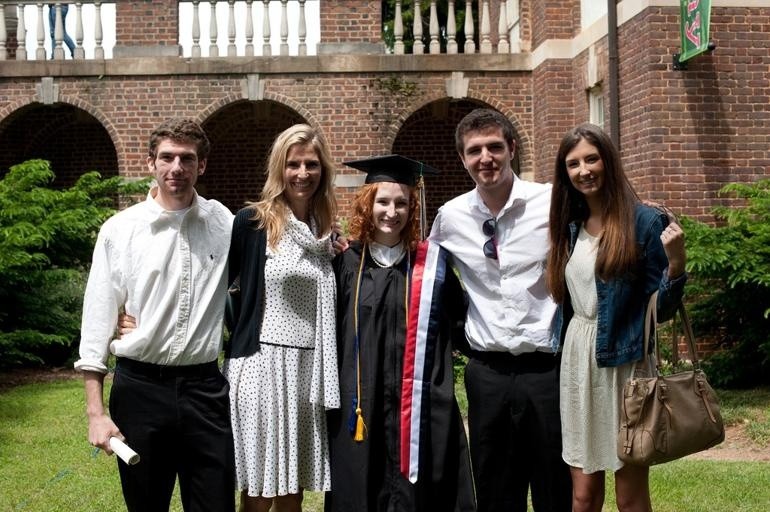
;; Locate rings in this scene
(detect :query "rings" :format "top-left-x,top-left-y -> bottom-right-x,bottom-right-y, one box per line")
668,228 -> 672,234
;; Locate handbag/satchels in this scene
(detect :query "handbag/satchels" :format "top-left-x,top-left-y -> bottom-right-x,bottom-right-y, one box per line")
614,288 -> 726,467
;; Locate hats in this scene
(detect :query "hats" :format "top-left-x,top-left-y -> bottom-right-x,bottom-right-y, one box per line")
341,154 -> 443,244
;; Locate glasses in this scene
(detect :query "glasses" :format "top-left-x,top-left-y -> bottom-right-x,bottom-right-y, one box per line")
481,216 -> 500,261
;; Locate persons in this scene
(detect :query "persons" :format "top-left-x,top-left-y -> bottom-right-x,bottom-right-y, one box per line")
544,121 -> 688,512
116,123 -> 345,512
332,106 -> 655,511
322,152 -> 477,512
73,116 -> 344,512
